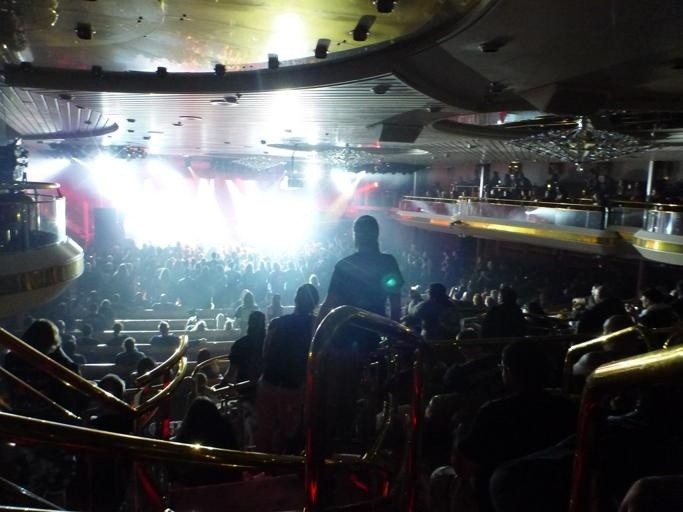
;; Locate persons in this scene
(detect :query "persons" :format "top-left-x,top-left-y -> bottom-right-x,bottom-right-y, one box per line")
1,167 -> 682,510
